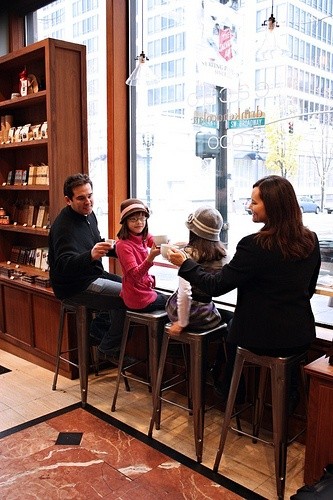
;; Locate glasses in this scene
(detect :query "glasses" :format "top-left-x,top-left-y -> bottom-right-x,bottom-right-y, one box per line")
125,217 -> 148,222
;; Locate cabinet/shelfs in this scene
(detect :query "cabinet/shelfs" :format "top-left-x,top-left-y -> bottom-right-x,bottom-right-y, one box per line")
0,35 -> 88,380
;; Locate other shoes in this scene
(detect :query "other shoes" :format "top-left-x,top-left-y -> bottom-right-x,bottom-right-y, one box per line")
209,360 -> 223,380
88,335 -> 101,347
96,348 -> 137,368
212,382 -> 245,407
287,391 -> 300,418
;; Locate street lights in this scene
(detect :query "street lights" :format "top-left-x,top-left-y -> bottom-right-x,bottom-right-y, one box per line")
139,131 -> 156,213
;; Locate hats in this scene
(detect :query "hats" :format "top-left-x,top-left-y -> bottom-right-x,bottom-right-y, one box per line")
119,199 -> 150,224
185,207 -> 223,242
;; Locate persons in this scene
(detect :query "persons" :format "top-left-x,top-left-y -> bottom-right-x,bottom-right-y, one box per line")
166,208 -> 228,379
48,173 -> 129,367
167,175 -> 321,414
115,199 -> 173,359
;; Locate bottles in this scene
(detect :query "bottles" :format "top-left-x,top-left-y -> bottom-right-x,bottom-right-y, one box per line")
0,208 -> 9,224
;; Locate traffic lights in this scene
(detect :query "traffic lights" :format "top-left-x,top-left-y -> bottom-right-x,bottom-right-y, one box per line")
288,121 -> 294,134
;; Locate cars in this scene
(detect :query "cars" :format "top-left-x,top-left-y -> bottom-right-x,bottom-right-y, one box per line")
298,197 -> 320,214
243,198 -> 253,216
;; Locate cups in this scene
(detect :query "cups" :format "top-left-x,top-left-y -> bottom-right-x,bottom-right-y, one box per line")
153,235 -> 168,247
160,244 -> 179,258
105,239 -> 116,249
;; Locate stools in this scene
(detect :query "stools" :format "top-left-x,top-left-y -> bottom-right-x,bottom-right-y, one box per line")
112,309 -> 193,430
53,300 -> 130,408
148,322 -> 242,462
214,345 -> 309,500
